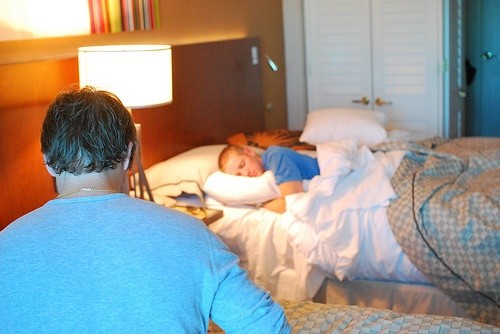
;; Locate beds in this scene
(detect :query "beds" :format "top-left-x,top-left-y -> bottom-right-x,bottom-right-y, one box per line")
0,34 -> 500,326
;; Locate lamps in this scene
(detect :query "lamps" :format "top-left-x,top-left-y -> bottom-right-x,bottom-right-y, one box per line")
77,44 -> 173,201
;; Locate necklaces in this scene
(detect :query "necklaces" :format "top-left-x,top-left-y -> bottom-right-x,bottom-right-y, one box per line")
54,187 -> 119,199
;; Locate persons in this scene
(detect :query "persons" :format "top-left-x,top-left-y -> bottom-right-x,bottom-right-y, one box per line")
0,85 -> 292,334
218,143 -> 320,214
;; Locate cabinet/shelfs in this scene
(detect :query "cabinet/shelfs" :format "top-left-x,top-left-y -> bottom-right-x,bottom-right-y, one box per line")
302,0 -> 467,140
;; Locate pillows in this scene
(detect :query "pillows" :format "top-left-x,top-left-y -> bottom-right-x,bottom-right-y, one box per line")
130,144 -> 267,199
298,108 -> 389,148
